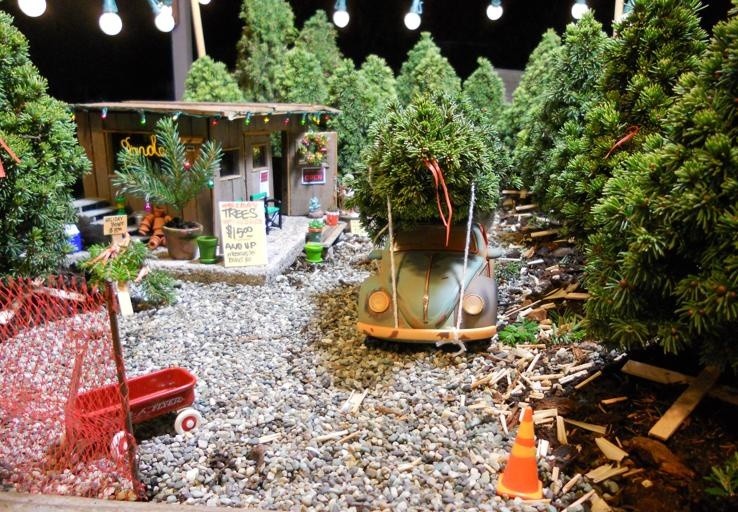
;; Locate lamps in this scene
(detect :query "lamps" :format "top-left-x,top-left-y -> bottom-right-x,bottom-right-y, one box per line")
18,0 -> 46,17
332,0 -> 350,29
149,0 -> 174,32
484,0 -> 502,20
569,0 -> 589,20
403,0 -> 424,29
97,0 -> 123,35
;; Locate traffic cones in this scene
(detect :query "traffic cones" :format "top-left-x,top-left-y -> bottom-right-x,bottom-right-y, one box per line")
496,406 -> 545,501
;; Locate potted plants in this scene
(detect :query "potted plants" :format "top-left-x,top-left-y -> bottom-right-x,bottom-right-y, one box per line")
325,206 -> 340,225
111,120 -> 223,260
308,219 -> 322,237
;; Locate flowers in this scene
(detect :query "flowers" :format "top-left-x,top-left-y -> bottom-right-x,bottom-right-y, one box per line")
296,129 -> 328,165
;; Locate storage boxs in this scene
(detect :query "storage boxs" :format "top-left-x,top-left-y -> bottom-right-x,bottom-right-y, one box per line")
62,232 -> 81,254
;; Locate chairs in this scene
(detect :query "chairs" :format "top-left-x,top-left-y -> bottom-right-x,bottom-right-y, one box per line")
251,192 -> 282,234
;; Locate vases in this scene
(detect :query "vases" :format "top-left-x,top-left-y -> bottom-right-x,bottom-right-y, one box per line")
303,243 -> 322,262
198,236 -> 219,263
